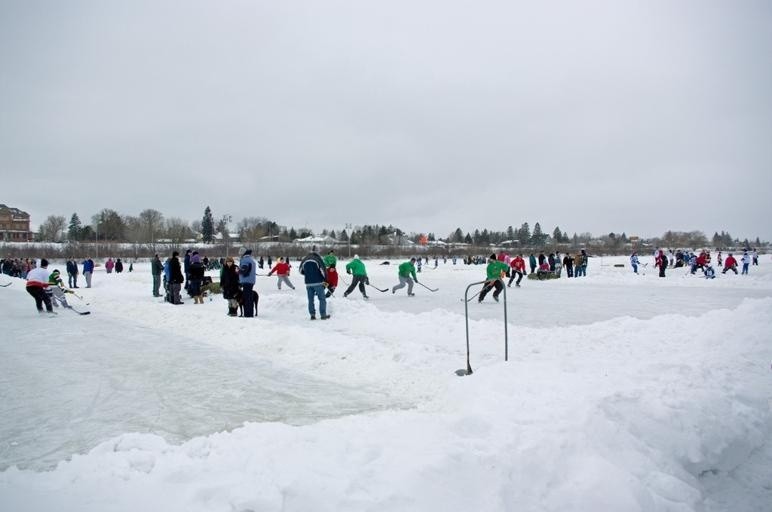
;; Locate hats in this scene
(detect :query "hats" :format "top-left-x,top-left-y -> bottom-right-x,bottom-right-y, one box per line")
224,256 -> 234,265
311,244 -> 320,253
239,247 -> 247,255
192,255 -> 200,262
353,254 -> 359,260
490,253 -> 496,261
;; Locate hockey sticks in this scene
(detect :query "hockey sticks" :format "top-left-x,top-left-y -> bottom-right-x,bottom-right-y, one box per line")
338,274 -> 349,285
415,280 -> 439,291
461,281 -> 495,302
363,282 -> 389,292
53,293 -> 91,315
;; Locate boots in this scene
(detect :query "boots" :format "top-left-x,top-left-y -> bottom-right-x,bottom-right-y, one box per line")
227,305 -> 237,316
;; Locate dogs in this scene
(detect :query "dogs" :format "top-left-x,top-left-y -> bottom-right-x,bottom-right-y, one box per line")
230,286 -> 259,318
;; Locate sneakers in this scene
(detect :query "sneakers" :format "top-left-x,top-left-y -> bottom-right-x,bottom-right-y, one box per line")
477,295 -> 500,305
391,290 -> 417,298
39,304 -> 73,318
307,291 -> 371,321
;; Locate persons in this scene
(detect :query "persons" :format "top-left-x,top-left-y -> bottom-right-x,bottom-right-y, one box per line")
105,259 -> 133,273
630,248 -> 759,279
392,258 -> 417,296
344,254 -> 370,299
151,247 -> 296,318
1,255 -> 95,313
416,255 -> 487,273
477,250 -> 588,304
299,246 -> 338,320
